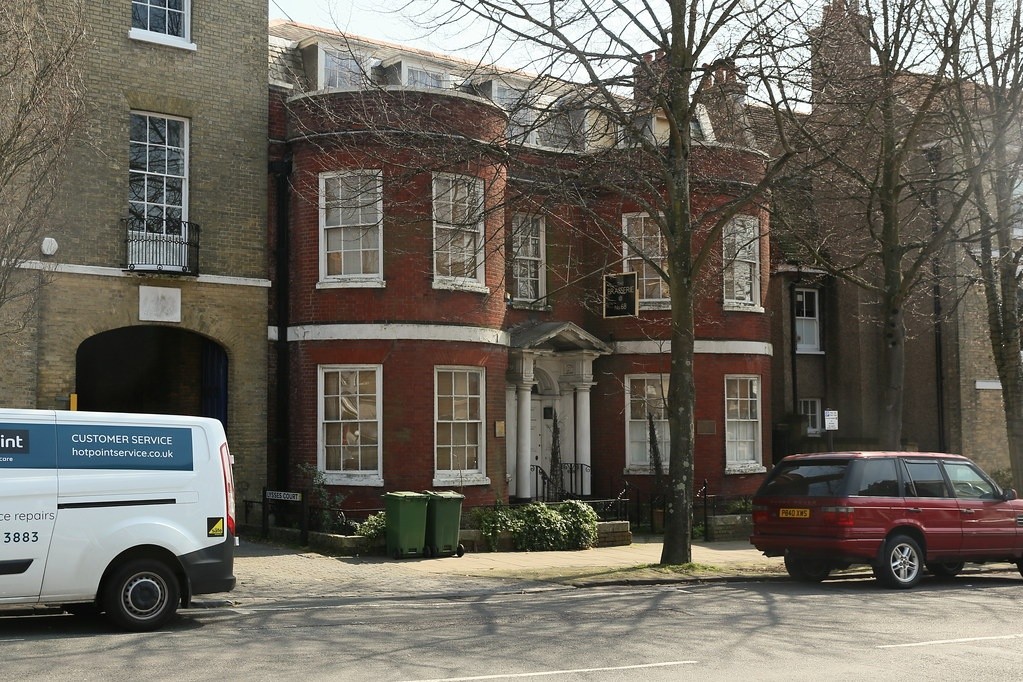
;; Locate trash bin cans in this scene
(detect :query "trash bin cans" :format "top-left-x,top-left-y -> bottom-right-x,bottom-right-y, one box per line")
420,489 -> 465,558
379,490 -> 430,560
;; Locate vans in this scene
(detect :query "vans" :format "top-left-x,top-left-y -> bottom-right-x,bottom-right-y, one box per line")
0,407 -> 241,636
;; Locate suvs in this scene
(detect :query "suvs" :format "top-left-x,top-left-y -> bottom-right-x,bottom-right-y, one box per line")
747,450 -> 1023,591
